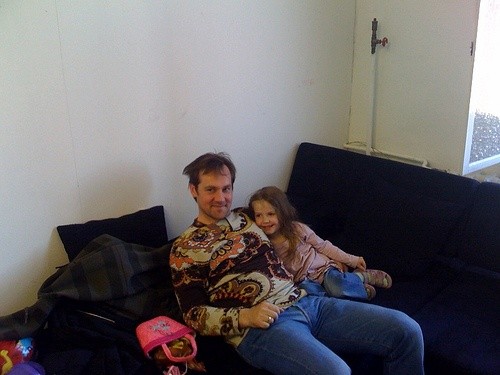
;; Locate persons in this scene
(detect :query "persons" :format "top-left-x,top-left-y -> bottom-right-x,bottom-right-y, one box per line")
169,154 -> 424,375
248,186 -> 391,300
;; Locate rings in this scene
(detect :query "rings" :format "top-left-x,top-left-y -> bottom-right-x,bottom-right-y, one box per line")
268,317 -> 271,322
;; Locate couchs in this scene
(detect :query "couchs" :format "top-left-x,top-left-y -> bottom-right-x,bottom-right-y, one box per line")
36,141 -> 500,375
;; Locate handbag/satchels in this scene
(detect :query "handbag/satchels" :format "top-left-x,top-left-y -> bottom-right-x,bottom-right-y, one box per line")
136,316 -> 197,363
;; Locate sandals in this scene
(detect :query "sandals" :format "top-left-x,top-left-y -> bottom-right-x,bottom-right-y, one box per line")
354,269 -> 392,289
